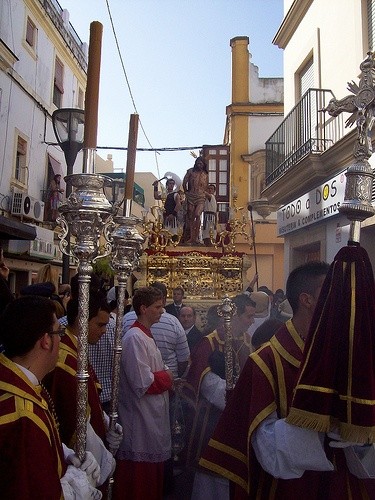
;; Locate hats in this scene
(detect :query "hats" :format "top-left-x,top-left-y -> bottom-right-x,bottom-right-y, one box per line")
277,299 -> 293,318
248,291 -> 271,319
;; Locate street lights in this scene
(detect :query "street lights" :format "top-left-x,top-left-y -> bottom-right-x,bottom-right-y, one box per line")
53,107 -> 86,297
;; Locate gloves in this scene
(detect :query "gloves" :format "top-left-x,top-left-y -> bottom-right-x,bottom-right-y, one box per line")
109,458 -> 116,477
105,423 -> 124,449
76,465 -> 97,488
67,451 -> 101,484
327,425 -> 367,448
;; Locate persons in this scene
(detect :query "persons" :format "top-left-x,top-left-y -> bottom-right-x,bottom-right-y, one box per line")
176,190 -> 186,244
153,179 -> 182,241
164,287 -> 185,319
206,274 -> 293,348
176,156 -> 210,247
43,174 -> 64,221
177,307 -> 204,353
112,286 -> 186,500
0,249 -> 138,315
39,292 -> 124,500
202,185 -> 218,246
194,261 -> 375,500
57,272 -> 116,416
173,295 -> 256,500
120,282 -> 190,496
0,297 -> 103,500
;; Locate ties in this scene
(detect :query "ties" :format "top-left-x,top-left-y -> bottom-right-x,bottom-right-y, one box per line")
176,307 -> 181,313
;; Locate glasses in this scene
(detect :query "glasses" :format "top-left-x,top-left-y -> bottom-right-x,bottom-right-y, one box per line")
48,327 -> 67,337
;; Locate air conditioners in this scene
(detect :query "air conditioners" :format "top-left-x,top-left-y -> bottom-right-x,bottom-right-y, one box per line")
11,193 -> 45,223
